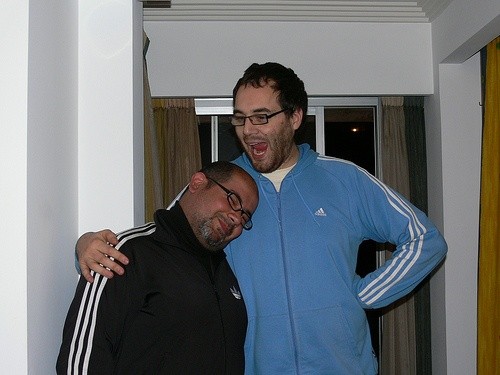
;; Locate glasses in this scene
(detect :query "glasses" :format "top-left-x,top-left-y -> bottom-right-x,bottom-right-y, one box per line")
228,106 -> 291,127
204,176 -> 254,231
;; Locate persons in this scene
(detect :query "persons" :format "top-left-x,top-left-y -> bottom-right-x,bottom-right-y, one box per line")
55,161 -> 260,375
74,62 -> 449,375
